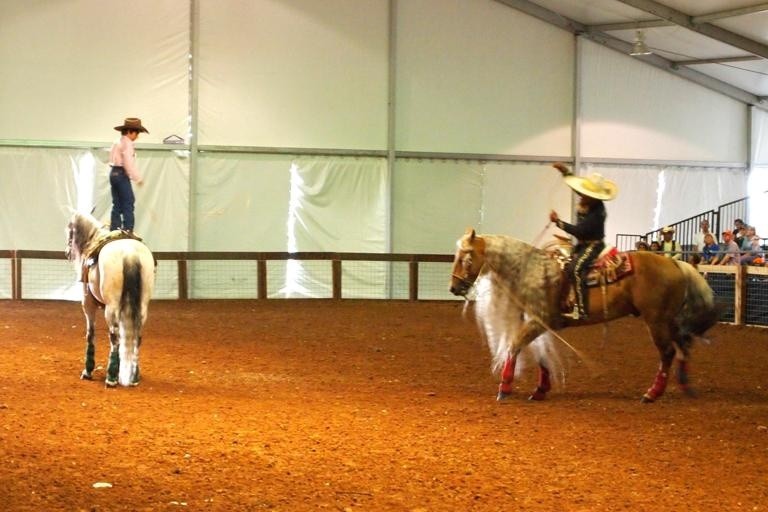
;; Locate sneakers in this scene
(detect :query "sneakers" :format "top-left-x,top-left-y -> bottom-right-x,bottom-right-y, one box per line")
124,229 -> 142,241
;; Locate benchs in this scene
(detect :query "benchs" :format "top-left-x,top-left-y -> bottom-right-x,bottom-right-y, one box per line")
699,246 -> 768,326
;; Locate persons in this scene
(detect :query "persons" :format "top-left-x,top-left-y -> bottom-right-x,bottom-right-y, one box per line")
549,162 -> 618,322
108,117 -> 150,240
633,218 -> 768,283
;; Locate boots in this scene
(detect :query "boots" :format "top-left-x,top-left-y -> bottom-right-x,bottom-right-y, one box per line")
561,305 -> 586,321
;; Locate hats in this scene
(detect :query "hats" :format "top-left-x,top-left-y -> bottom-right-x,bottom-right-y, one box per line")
661,227 -> 674,233
564,173 -> 619,200
113,118 -> 150,134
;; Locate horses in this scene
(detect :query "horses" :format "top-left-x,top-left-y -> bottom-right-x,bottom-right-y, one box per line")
448,228 -> 728,407
64,202 -> 155,388
